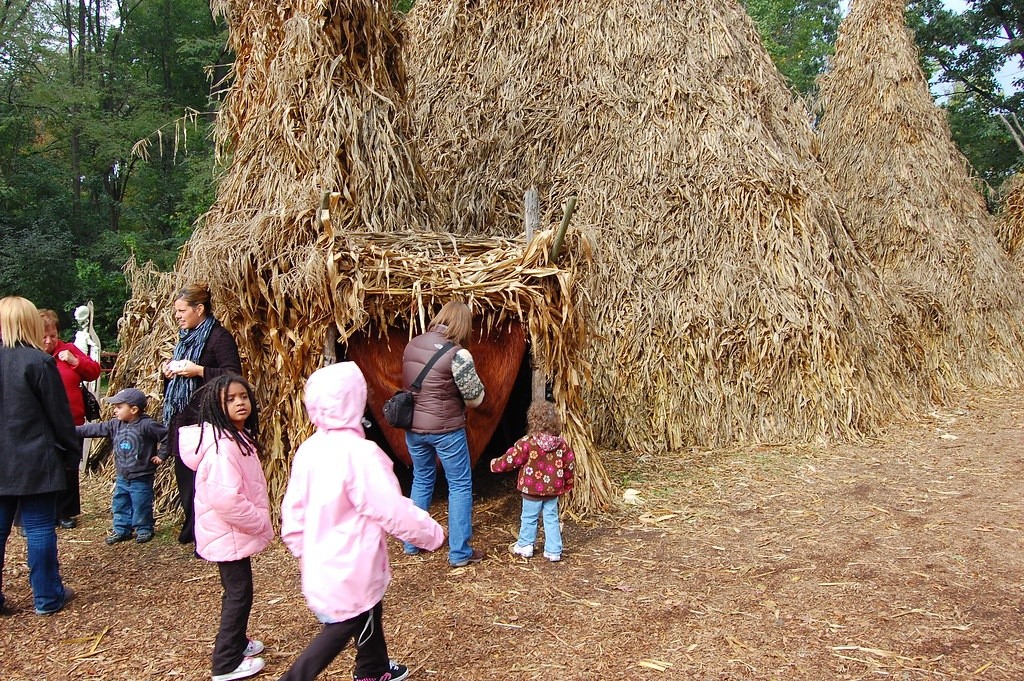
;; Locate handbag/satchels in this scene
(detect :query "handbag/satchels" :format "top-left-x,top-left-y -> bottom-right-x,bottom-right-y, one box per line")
383,390 -> 413,429
80,386 -> 103,423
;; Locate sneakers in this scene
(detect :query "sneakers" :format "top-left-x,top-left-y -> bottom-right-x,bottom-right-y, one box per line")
514,543 -> 534,557
106,530 -> 132,544
353,662 -> 409,681
543,551 -> 560,561
403,549 -> 419,555
137,532 -> 156,543
451,549 -> 484,567
212,656 -> 264,681
243,637 -> 264,657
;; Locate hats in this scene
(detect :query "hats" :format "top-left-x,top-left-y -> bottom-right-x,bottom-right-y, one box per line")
104,388 -> 147,410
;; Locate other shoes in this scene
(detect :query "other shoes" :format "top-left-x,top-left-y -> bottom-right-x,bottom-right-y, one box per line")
58,517 -> 75,528
21,525 -> 27,537
0,606 -> 19,618
36,589 -> 75,615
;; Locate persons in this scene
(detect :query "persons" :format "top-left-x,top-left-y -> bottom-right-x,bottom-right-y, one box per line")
74,388 -> 169,544
491,399 -> 575,561
37,308 -> 100,529
276,360 -> 445,681
160,283 -> 243,558
401,300 -> 486,567
0,295 -> 77,616
178,374 -> 275,681
